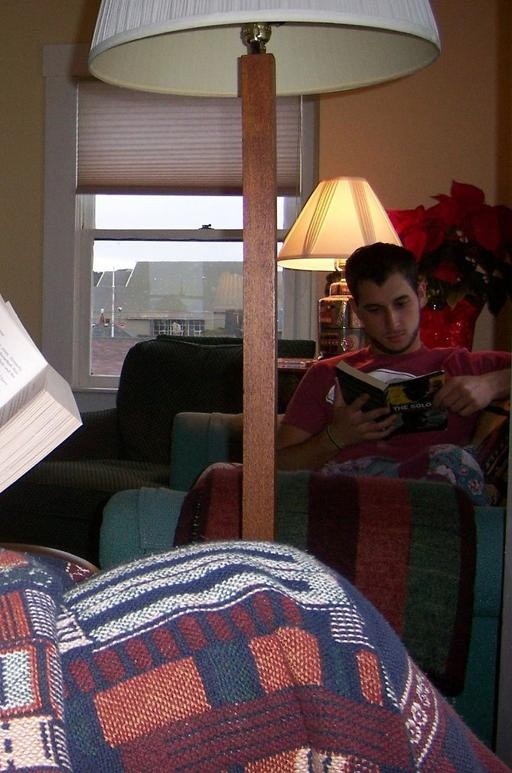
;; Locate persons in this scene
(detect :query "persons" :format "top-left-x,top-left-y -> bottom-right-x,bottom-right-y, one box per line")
173,243 -> 512,551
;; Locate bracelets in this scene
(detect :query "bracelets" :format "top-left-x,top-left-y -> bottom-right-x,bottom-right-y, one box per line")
323,425 -> 344,454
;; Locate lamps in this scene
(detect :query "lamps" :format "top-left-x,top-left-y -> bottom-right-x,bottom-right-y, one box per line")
85,1 -> 442,543
283,173 -> 406,339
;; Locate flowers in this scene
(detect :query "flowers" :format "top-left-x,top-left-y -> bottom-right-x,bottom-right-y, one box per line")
379,180 -> 510,318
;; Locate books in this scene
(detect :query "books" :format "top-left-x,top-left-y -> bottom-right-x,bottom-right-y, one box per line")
336,356 -> 450,443
0,295 -> 83,497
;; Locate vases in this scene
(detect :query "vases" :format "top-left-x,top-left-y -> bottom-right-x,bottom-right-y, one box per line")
416,278 -> 489,352
